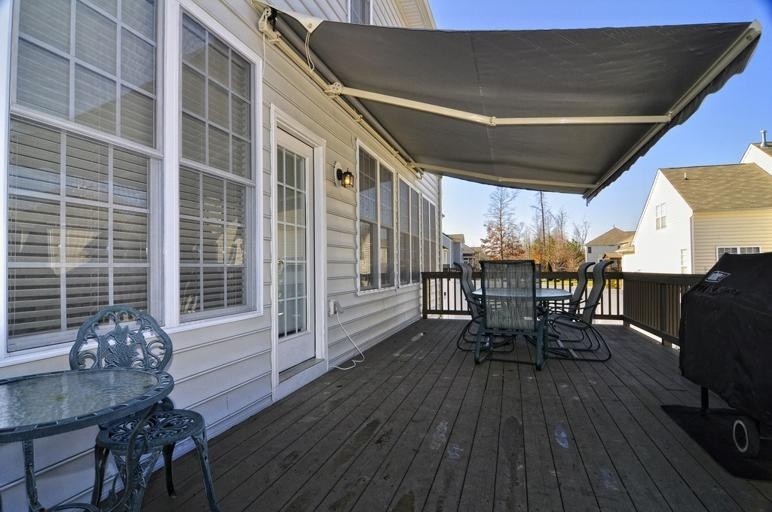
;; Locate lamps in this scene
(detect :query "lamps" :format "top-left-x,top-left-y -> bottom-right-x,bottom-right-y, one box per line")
336,166 -> 355,191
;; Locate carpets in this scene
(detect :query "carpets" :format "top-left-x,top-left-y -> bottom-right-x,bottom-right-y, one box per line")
660,403 -> 772,482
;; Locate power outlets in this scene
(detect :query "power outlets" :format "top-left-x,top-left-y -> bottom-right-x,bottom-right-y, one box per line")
328,298 -> 340,318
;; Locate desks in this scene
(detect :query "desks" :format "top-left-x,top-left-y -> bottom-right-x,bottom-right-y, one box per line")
0,365 -> 175,512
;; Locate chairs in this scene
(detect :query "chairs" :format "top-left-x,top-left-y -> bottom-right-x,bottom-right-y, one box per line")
60,300 -> 223,512
443,249 -> 619,374
